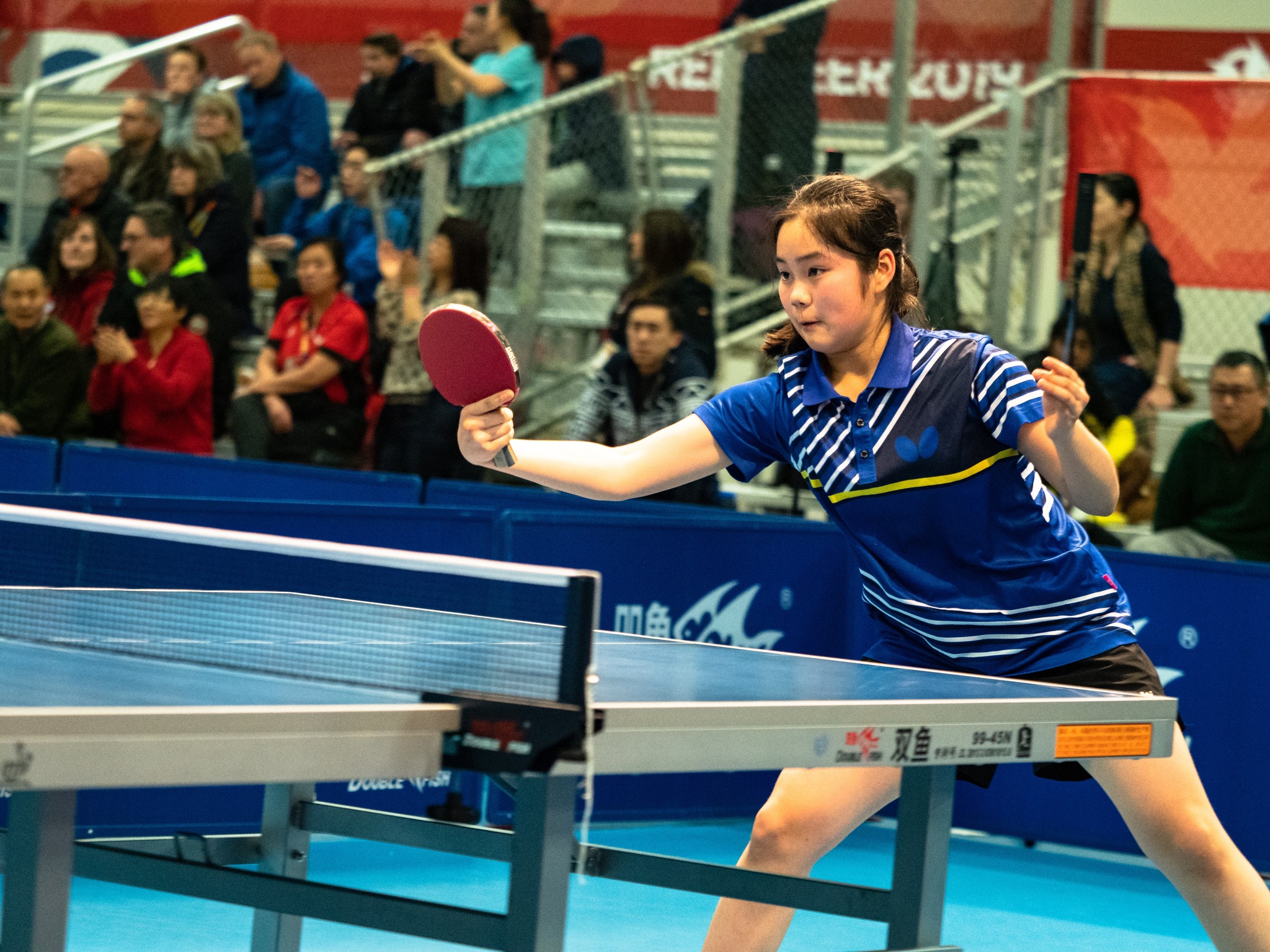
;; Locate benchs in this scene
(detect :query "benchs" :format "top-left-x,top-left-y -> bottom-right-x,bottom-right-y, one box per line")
1,87 -> 1270,545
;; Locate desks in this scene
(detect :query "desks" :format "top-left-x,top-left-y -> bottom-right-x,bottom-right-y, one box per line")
0,577 -> 1180,952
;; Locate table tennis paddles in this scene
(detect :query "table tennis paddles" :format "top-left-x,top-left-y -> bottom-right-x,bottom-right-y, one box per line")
417,301 -> 522,469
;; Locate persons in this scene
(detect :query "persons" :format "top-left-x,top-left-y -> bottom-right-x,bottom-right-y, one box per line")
684,0 -> 828,219
4,3 -> 636,480
584,210 -> 717,378
1116,349 -> 1270,565
456,168 -> 1269,952
573,285 -> 722,508
1061,170 -> 1197,412
866,165 -> 916,251
1019,308 -> 1148,514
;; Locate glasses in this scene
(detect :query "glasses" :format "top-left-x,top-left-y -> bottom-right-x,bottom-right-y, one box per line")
1209,382 -> 1262,397
